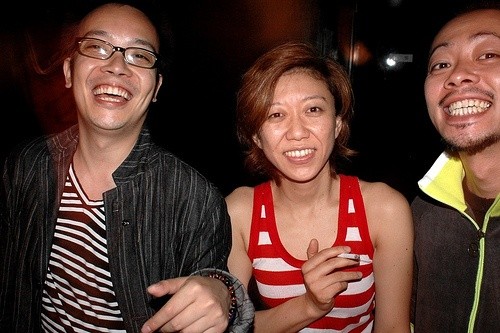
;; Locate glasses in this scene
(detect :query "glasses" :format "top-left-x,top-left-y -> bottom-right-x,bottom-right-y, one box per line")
70,36 -> 162,69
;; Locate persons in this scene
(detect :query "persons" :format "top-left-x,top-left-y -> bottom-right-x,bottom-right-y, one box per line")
407,0 -> 499,333
221,40 -> 417,333
0,0 -> 255,333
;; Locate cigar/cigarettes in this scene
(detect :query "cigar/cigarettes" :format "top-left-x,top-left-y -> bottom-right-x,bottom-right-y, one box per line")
336,253 -> 360,259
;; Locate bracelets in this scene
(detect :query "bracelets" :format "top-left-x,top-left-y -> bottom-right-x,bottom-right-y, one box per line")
203,271 -> 237,324
